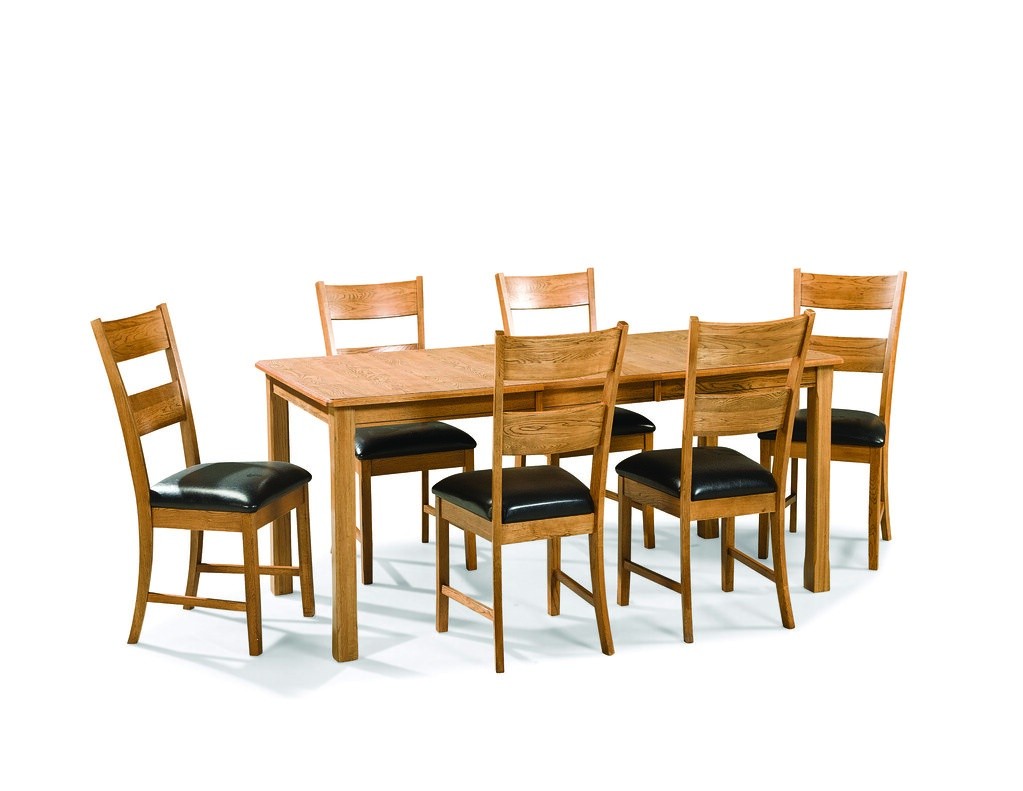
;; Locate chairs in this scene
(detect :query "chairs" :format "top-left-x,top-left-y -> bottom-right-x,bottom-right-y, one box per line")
756,269 -> 908,571
616,308 -> 818,643
431,320 -> 631,672
90,304 -> 316,658
494,266 -> 657,548
315,275 -> 479,586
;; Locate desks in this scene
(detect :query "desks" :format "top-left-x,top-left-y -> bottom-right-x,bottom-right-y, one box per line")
254,327 -> 844,666
